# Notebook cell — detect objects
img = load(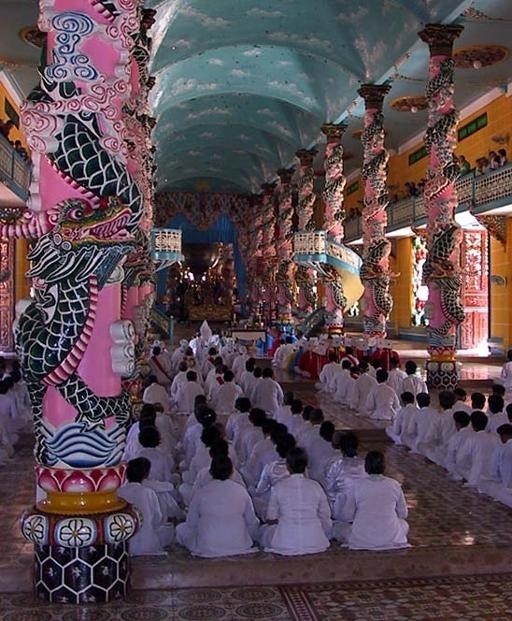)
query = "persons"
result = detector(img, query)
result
[273,333,311,370]
[149,340,172,382]
[225,397,333,556]
[385,384,510,509]
[171,339,206,414]
[143,375,174,415]
[0,356,33,466]
[180,395,260,555]
[314,347,428,420]
[268,393,413,551]
[411,298,426,328]
[495,351,511,388]
[120,402,183,555]
[190,334,284,415]
